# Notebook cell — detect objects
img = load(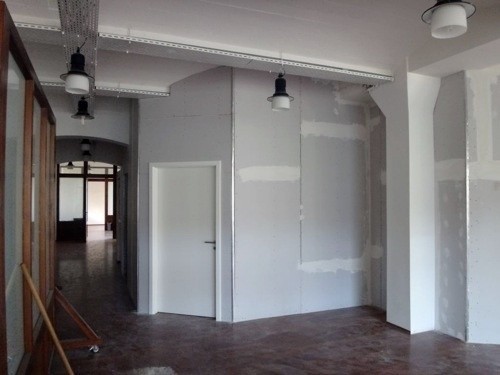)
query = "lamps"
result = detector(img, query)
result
[267,50,294,110]
[59,39,93,94]
[80,139,92,157]
[420,0,476,39]
[71,97,95,125]
